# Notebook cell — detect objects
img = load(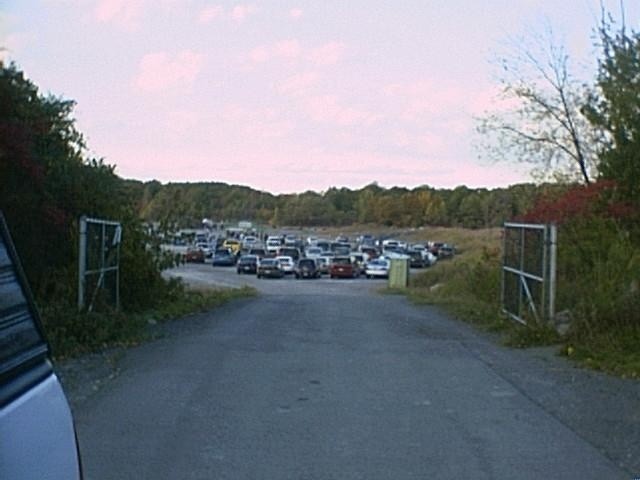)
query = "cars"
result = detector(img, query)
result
[163,217,456,281]
